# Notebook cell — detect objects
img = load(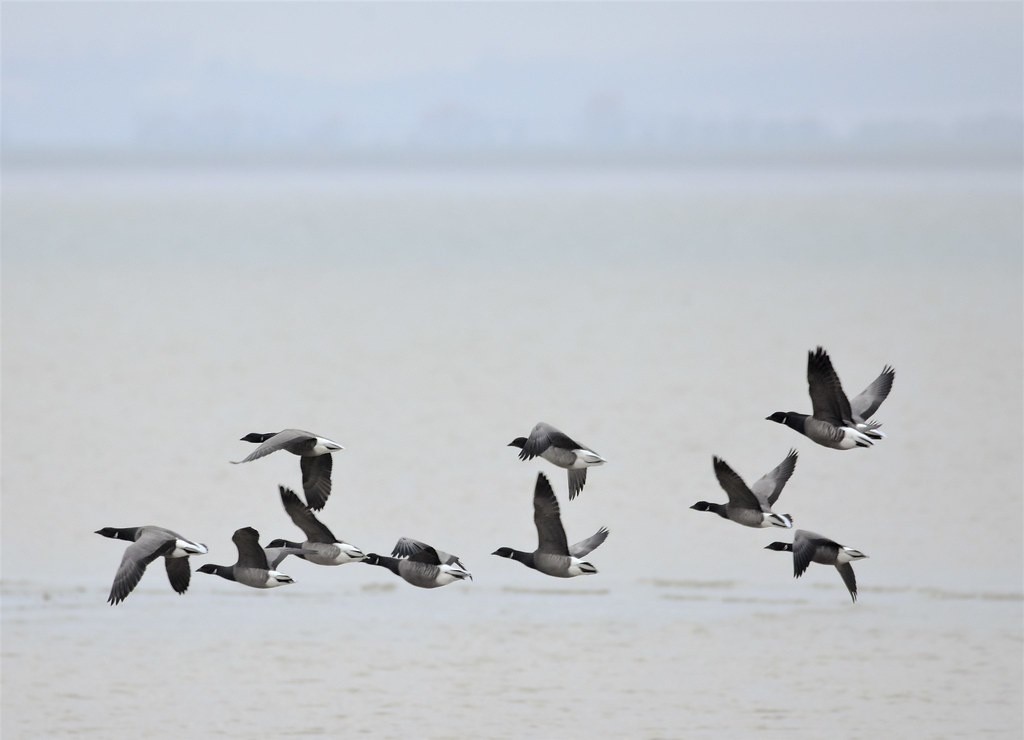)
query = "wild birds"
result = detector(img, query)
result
[688,447,799,529]
[763,528,870,604]
[506,420,608,501]
[93,428,474,606]
[764,346,894,451]
[491,471,610,579]
